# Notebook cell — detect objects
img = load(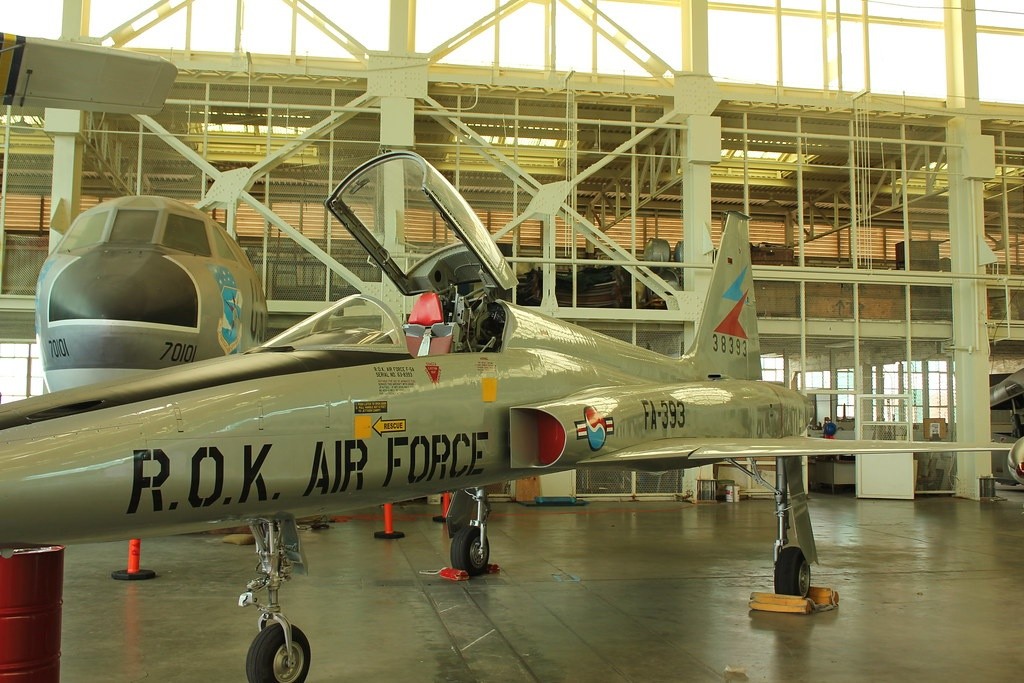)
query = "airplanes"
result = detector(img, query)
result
[0,151,1024,598]
[33,194,269,395]
